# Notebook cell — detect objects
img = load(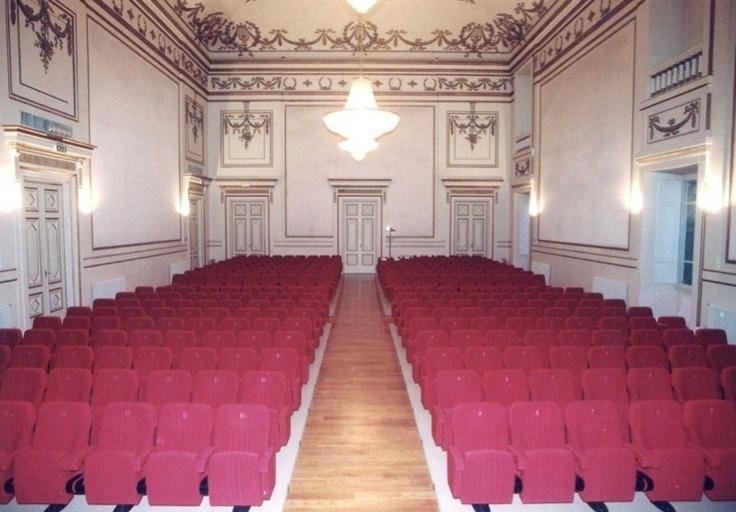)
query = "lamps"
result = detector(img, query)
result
[322,0,399,162]
